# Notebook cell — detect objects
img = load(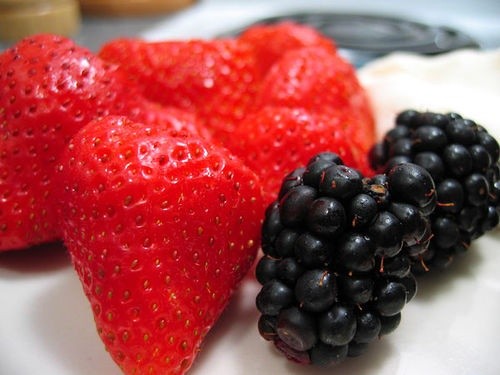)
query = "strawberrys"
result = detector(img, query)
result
[0,21,379,375]
